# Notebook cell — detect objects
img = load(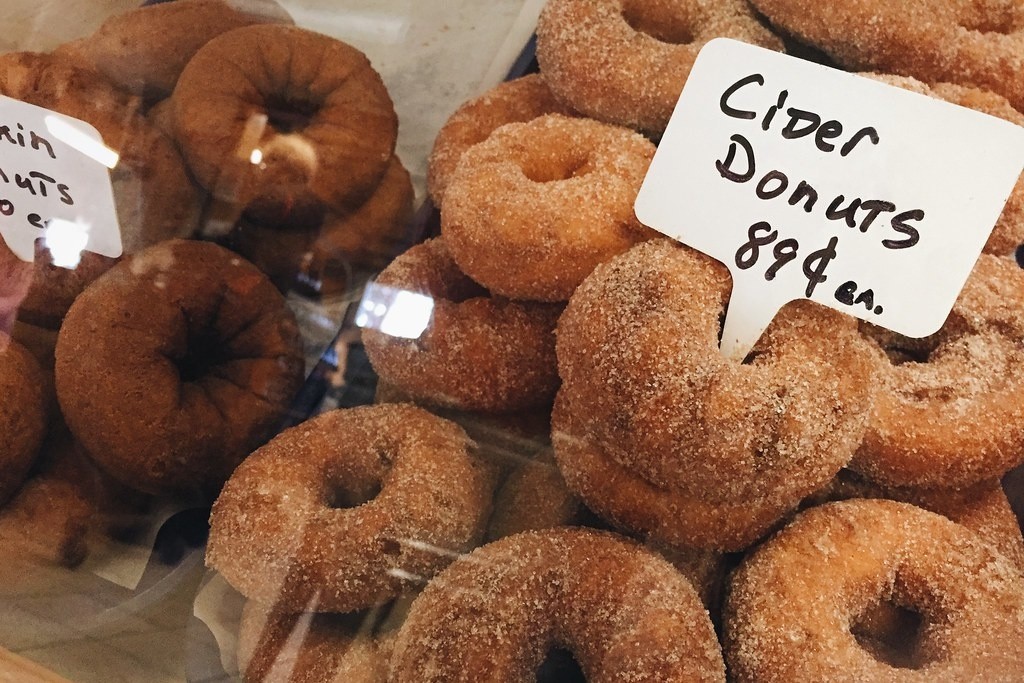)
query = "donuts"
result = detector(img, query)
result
[0,0,1024,683]
